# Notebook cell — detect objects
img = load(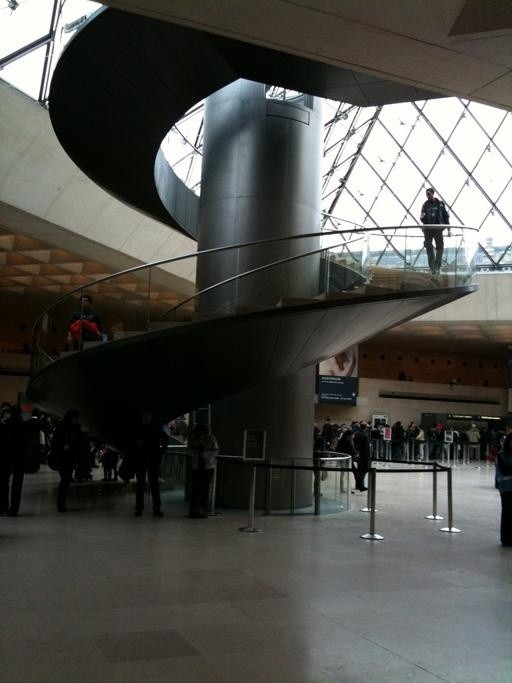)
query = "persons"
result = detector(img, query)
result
[0,401,221,522]
[420,186,448,273]
[318,349,356,377]
[496,432,512,547]
[69,293,108,348]
[315,415,512,490]
